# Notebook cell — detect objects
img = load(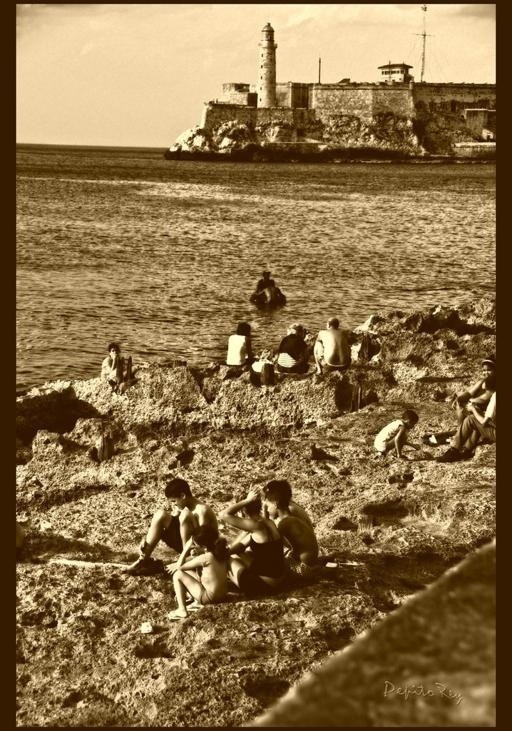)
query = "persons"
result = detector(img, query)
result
[121,478,320,623]
[251,279,282,306]
[373,410,420,459]
[101,343,123,377]
[435,377,495,463]
[445,355,496,444]
[255,270,274,291]
[315,318,351,376]
[227,323,257,377]
[277,324,313,374]
